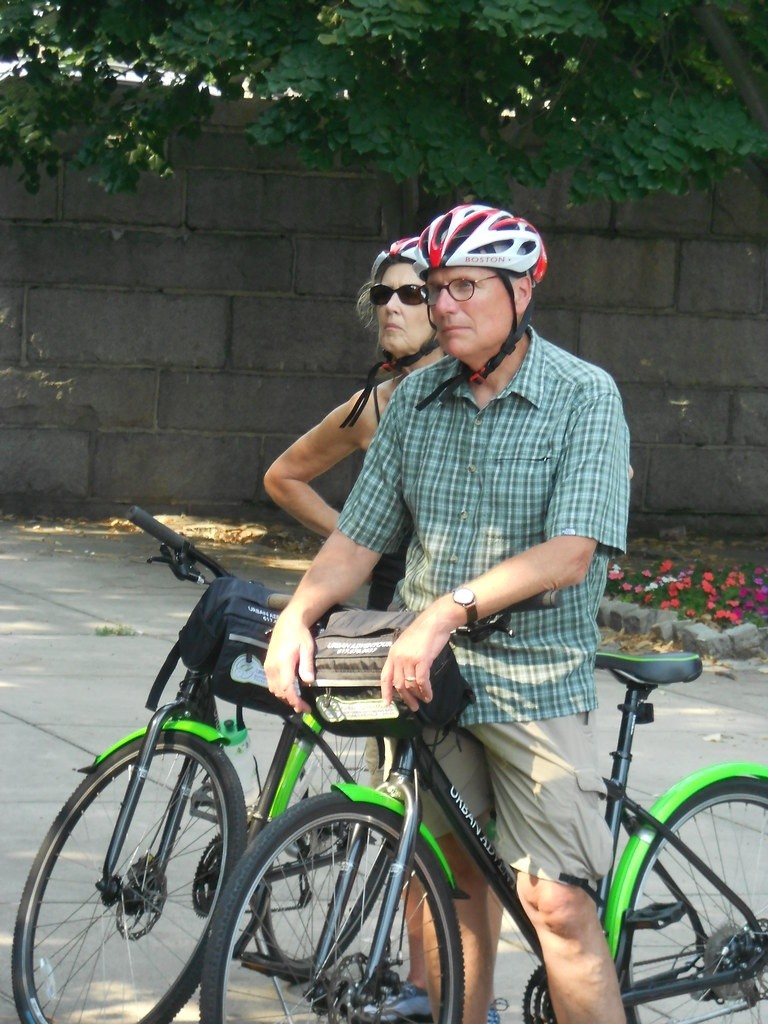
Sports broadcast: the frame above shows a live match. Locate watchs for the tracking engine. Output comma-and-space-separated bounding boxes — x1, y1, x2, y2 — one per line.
452, 586, 478, 627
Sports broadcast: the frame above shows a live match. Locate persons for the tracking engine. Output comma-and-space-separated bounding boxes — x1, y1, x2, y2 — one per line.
264, 237, 507, 1024
265, 205, 633, 1024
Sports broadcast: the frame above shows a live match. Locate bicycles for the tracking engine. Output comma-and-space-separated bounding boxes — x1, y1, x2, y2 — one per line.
197, 587, 768, 1024
9, 504, 394, 1024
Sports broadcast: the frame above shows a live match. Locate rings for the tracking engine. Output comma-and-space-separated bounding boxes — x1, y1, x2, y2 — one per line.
404, 677, 415, 681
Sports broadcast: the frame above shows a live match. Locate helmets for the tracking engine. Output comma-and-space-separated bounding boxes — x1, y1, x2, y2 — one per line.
370, 235, 420, 285
414, 203, 548, 288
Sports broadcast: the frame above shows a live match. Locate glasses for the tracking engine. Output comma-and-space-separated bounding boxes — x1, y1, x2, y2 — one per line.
368, 284, 430, 305
413, 275, 500, 306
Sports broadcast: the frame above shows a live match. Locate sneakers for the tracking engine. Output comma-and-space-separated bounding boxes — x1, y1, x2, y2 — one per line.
354, 980, 432, 1024
